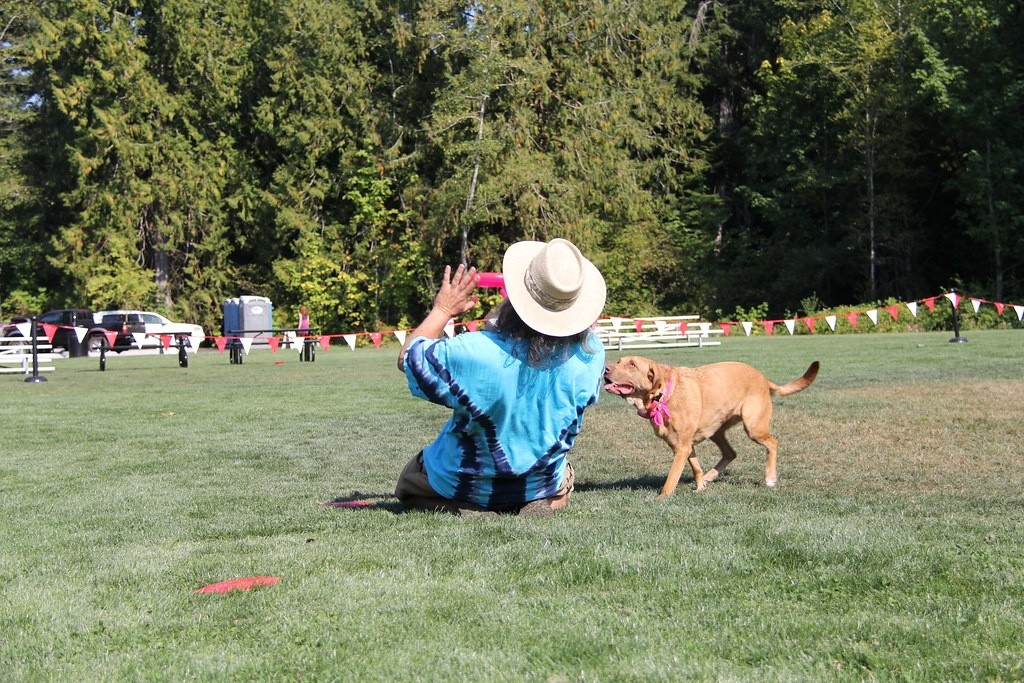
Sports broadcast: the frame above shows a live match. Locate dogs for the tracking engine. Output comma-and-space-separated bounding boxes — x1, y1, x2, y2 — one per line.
601, 355, 822, 498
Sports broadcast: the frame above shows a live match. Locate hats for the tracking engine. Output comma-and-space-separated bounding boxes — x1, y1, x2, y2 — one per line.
502, 238, 607, 337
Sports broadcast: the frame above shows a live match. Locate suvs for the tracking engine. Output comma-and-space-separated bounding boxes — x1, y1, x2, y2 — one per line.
93, 311, 206, 354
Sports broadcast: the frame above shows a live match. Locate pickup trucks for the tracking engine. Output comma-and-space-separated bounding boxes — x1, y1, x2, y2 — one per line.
2, 309, 134, 354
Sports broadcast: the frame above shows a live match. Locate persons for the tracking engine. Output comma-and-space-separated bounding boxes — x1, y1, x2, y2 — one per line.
396, 238, 607, 513
297, 306, 309, 337
